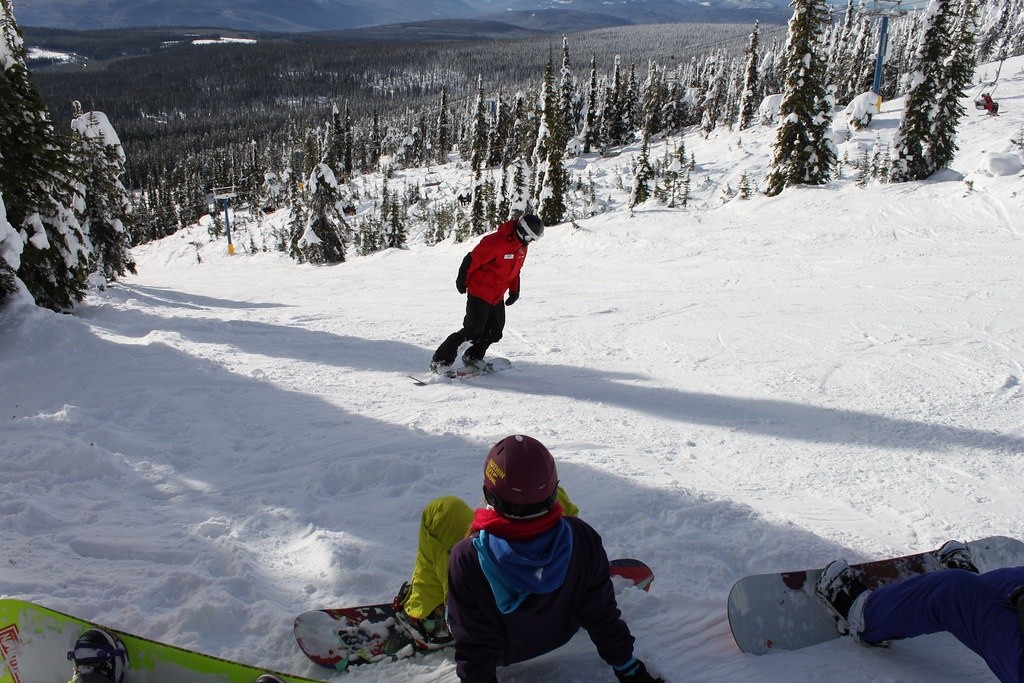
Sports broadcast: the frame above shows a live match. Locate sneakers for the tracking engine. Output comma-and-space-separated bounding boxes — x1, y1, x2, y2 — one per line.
73, 628, 123, 683
256, 674, 285, 683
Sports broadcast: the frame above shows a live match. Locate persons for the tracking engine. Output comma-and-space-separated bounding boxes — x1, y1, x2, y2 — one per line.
818, 539, 1023, 683
396, 433, 667, 683
429, 214, 545, 381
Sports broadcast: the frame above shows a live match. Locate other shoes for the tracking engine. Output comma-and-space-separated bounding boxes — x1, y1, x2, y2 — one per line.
819, 562, 891, 647
392, 581, 447, 641
462, 353, 493, 371
938, 539, 979, 574
430, 361, 456, 378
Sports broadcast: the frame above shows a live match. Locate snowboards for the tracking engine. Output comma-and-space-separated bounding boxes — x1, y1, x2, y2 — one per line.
0, 600, 328, 683
727, 535, 1024, 658
407, 356, 514, 385
292, 556, 656, 668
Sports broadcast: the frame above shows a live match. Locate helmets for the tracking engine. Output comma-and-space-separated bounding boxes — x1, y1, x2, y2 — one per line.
516, 215, 544, 245
484, 435, 559, 517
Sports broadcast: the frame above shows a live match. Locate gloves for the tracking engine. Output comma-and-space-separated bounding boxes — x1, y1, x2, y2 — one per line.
505, 292, 519, 306
613, 659, 664, 683
456, 275, 467, 294
